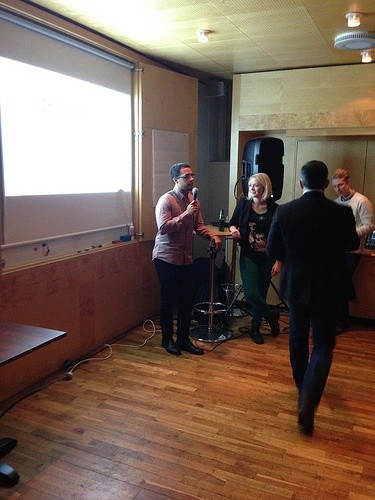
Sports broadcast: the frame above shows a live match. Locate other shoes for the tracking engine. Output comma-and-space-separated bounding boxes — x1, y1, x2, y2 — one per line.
269, 310, 280, 337
250, 329, 264, 344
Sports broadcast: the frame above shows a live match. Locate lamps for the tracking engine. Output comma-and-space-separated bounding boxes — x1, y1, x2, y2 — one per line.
197, 30, 212, 42
345, 13, 363, 27
333, 32, 375, 50
360, 51, 372, 63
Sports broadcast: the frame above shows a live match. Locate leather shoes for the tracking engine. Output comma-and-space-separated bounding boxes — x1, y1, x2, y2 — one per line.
162, 336, 181, 355
176, 337, 203, 355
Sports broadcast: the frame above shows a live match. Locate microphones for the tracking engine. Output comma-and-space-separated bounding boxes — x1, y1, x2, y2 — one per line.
192, 186, 199, 201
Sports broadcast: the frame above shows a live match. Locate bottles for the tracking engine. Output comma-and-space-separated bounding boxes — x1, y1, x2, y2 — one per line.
219, 209, 225, 232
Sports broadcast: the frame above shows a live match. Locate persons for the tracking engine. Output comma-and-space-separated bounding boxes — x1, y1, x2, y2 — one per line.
228, 173, 280, 345
265, 160, 360, 435
152, 162, 223, 355
332, 169, 375, 336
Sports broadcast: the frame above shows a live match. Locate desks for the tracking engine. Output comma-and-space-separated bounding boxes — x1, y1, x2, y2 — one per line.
191, 225, 232, 343
0, 320, 67, 486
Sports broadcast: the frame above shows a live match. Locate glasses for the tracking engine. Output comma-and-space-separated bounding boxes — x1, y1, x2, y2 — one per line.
178, 173, 196, 179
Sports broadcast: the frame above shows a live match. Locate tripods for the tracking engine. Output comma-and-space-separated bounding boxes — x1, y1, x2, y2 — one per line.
225, 274, 290, 320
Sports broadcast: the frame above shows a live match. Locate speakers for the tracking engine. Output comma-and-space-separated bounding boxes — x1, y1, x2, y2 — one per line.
242, 136, 285, 201
